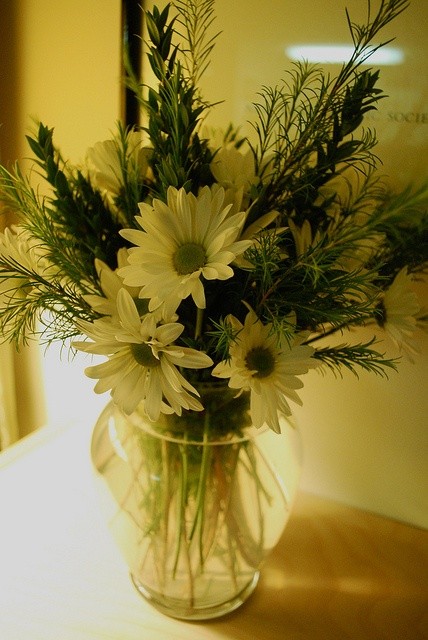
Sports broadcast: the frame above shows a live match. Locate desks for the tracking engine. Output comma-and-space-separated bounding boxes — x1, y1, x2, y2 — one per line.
0, 416, 426, 639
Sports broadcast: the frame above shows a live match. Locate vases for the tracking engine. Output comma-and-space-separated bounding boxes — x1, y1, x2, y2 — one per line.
91, 385, 299, 625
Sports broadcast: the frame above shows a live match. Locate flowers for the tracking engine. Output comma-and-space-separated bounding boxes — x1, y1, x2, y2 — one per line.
0, 0, 428, 609
